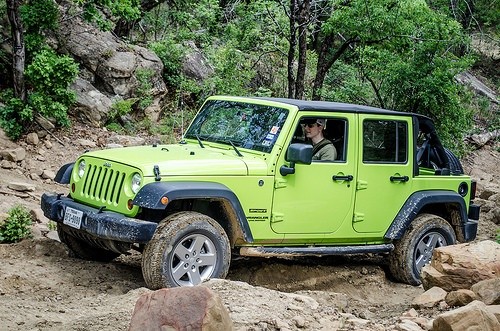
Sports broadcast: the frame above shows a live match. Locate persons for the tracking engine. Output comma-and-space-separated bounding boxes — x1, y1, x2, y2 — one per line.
297, 116, 339, 161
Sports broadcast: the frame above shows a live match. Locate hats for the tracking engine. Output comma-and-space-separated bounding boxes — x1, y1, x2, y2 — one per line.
300, 118, 325, 126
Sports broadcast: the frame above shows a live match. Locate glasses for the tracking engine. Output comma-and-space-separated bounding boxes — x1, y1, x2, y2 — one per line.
304, 124, 317, 128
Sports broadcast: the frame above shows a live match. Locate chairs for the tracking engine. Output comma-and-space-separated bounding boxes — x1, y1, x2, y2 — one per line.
325, 119, 344, 160
385, 127, 405, 160
294, 126, 304, 143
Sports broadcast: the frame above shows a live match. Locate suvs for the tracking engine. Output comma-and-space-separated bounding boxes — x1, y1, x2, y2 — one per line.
40, 94, 482, 292
